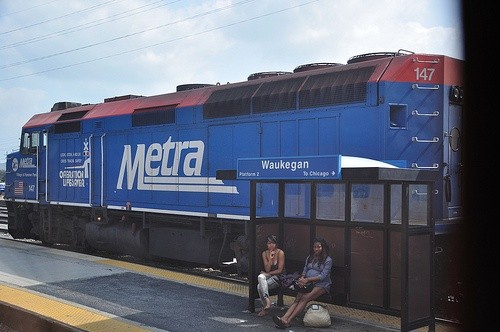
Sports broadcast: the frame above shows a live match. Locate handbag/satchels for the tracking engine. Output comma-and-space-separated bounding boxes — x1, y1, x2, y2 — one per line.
303, 304, 331, 327
294, 281, 313, 292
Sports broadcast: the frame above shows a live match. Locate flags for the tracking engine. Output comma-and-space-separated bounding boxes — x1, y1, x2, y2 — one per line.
13, 180, 23, 196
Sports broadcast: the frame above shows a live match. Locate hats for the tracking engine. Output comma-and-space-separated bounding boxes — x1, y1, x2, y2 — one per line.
267, 234, 276, 242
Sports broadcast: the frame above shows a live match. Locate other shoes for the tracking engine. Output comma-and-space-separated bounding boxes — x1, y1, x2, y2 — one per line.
256, 310, 268, 317
264, 301, 271, 311
272, 315, 291, 329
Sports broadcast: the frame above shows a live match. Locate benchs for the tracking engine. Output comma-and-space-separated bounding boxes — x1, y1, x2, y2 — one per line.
268, 260, 350, 303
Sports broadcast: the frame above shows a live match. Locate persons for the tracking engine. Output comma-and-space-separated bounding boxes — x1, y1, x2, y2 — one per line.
273, 239, 332, 328
257, 234, 288, 317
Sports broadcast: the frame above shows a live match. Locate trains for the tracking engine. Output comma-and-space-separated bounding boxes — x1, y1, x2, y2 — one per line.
2, 48, 465, 277
